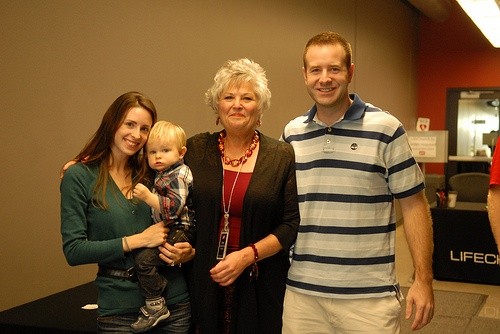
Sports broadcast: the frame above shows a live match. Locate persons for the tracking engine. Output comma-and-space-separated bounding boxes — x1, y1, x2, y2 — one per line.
278, 32, 435, 334
60, 91, 197, 334
61, 58, 300, 334
128, 121, 198, 332
486, 134, 499, 251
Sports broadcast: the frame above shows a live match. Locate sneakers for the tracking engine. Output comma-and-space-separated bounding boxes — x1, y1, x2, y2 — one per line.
129, 302, 170, 332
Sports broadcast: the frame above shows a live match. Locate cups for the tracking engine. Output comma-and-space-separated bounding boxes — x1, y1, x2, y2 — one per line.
447, 191, 458, 208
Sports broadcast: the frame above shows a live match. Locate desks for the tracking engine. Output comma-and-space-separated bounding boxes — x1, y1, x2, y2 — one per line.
429, 199, 500, 286
0, 278, 99, 334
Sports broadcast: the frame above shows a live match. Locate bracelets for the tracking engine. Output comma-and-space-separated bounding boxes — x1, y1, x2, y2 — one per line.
249, 243, 259, 263
124, 236, 132, 252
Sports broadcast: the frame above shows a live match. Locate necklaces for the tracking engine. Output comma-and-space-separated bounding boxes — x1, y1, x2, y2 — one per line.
217, 130, 260, 167
214, 140, 254, 259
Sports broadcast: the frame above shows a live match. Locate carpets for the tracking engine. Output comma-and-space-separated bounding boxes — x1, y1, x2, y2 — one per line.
399, 285, 500, 334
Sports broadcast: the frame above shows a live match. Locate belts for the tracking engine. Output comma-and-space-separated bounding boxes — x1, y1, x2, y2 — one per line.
97, 266, 139, 279
